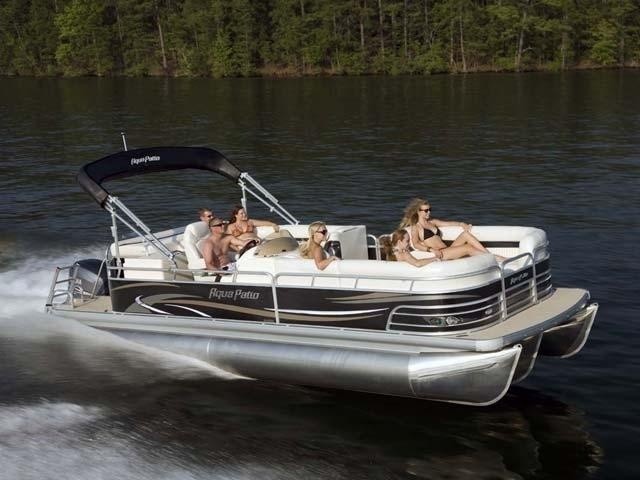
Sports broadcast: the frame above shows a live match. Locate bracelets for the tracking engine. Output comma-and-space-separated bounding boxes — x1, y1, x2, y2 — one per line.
427, 247, 432, 252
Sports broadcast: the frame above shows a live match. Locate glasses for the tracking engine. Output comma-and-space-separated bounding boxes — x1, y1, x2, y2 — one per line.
211, 223, 225, 226
202, 213, 214, 219
317, 230, 327, 236
419, 207, 431, 214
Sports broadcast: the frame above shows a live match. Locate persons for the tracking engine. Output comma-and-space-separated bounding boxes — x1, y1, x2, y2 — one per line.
299, 221, 340, 270
202, 217, 252, 276
382, 229, 441, 266
199, 207, 214, 222
399, 197, 506, 262
225, 206, 279, 253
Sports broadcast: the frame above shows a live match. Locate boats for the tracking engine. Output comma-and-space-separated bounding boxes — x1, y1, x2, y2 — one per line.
46, 130, 599, 408
